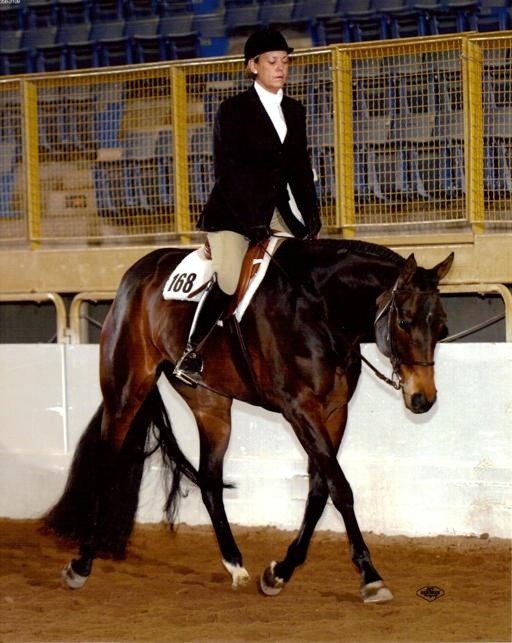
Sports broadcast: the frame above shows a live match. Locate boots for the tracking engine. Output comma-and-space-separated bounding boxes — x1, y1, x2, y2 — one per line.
175, 272, 237, 388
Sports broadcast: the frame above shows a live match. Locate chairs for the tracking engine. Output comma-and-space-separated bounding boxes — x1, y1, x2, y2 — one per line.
0, 0, 512, 219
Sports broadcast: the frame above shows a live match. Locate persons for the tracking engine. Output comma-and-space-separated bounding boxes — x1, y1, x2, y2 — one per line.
173, 27, 323, 385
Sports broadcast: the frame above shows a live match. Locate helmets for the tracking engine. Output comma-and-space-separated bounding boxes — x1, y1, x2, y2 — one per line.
244, 29, 293, 63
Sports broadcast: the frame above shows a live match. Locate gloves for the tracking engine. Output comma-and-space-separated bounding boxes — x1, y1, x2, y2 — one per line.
247, 225, 270, 241
307, 212, 322, 238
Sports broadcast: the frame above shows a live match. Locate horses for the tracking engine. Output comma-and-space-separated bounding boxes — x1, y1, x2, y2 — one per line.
35, 238, 455, 605
